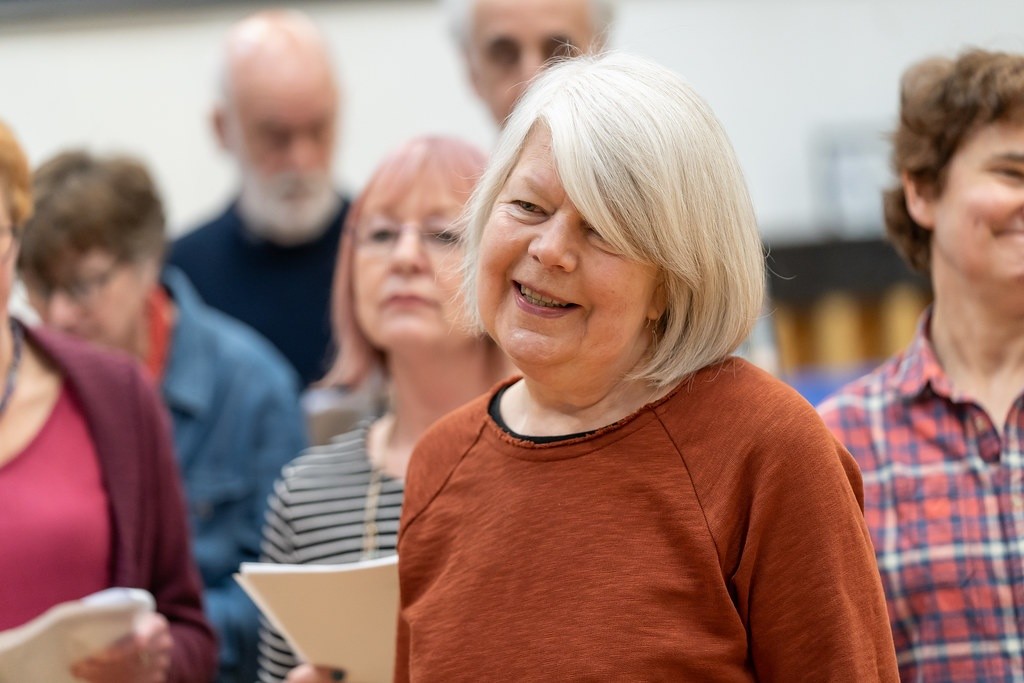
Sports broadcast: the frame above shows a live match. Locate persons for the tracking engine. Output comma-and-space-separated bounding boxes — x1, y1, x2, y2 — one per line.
12, 151, 312, 682
165, 8, 357, 403
2, 121, 219, 682
813, 45, 1024, 682
444, 1, 620, 133
230, 136, 517, 680
393, 54, 901, 683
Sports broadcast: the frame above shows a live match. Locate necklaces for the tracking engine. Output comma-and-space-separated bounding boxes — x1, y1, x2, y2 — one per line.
362, 410, 395, 561
0, 314, 25, 410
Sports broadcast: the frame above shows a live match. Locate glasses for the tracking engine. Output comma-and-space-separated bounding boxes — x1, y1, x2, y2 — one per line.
361, 227, 460, 249
17, 250, 141, 301
